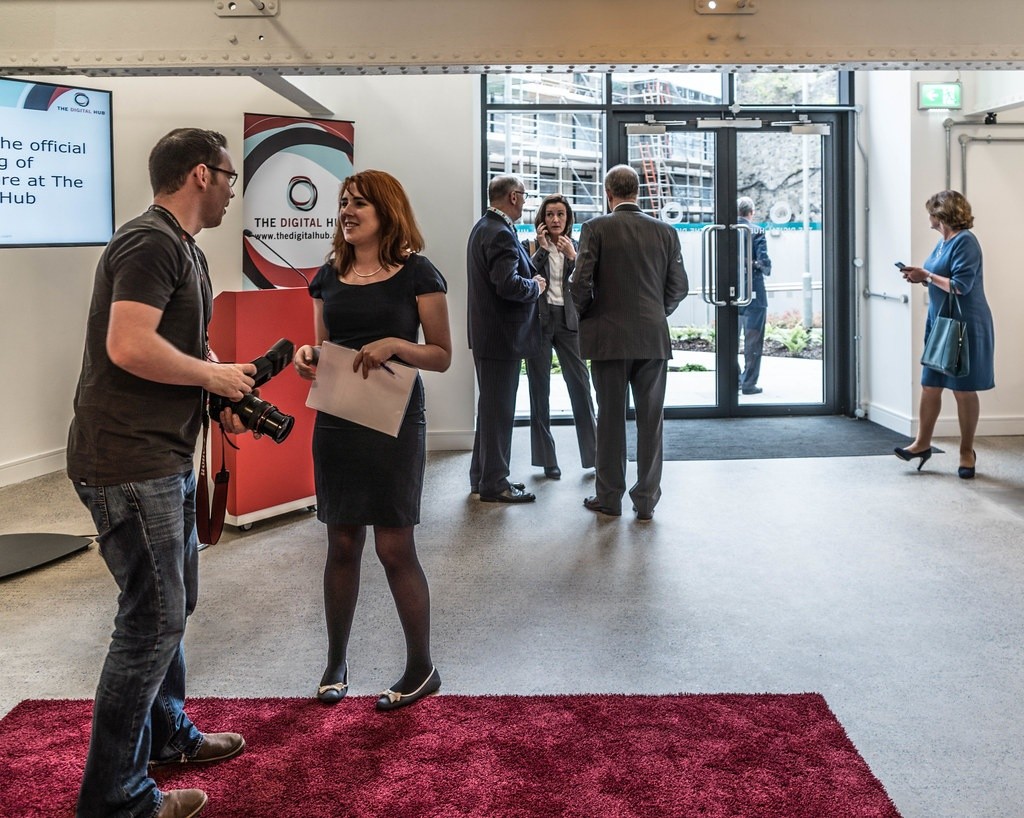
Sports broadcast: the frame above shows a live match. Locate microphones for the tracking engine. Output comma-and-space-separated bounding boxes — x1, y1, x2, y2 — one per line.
243, 228, 309, 286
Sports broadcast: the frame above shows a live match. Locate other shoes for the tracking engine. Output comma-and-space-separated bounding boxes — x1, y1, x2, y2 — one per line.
583, 496, 621, 516
637, 509, 655, 522
742, 385, 762, 394
162, 732, 246, 763
317, 659, 349, 703
156, 788, 206, 818
376, 665, 441, 710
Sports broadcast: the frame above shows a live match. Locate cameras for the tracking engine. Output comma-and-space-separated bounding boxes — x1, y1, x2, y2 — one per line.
212, 337, 296, 444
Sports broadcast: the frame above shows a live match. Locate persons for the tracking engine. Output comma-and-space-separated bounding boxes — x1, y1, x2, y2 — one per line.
521, 194, 597, 478
569, 164, 689, 519
894, 190, 996, 479
292, 170, 452, 710
66, 128, 260, 818
737, 197, 772, 395
467, 176, 546, 503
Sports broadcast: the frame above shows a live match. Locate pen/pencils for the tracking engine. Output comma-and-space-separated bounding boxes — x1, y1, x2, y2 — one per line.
381, 363, 395, 375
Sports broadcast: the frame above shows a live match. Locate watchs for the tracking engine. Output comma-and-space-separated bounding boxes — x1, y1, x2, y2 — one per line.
926, 273, 933, 283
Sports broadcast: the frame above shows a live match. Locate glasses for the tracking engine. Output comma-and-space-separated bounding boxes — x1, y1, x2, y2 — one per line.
194, 165, 238, 187
509, 191, 528, 199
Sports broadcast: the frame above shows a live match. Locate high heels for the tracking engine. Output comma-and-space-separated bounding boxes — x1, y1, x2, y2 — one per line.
958, 450, 976, 479
894, 447, 932, 471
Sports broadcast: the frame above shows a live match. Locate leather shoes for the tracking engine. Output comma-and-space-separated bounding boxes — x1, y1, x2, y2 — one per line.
544, 465, 561, 479
471, 482, 525, 494
480, 485, 536, 502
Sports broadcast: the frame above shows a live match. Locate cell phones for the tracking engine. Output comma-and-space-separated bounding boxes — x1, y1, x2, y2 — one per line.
895, 262, 905, 269
542, 220, 549, 235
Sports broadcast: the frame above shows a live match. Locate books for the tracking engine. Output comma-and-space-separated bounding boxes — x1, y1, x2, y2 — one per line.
305, 341, 418, 438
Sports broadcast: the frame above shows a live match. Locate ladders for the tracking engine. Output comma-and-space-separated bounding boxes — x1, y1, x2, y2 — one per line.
642, 81, 672, 207
640, 142, 661, 219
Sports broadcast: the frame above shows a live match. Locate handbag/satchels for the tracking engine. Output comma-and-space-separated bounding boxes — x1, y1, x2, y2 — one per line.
920, 279, 970, 379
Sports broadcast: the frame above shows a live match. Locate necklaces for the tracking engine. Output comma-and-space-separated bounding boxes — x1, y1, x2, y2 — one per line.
352, 260, 386, 277
937, 232, 954, 257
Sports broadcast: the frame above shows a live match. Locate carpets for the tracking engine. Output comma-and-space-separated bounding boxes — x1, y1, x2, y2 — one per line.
597, 414, 946, 461
0, 692, 903, 818
